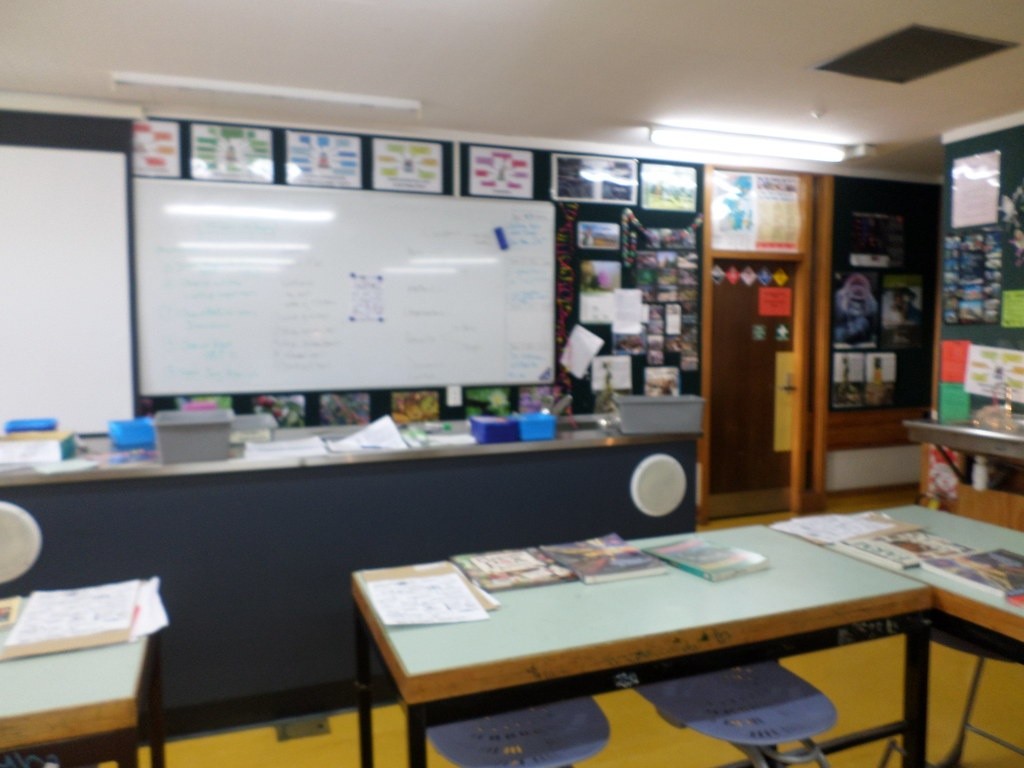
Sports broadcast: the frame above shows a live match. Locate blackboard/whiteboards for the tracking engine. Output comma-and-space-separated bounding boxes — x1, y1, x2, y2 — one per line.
127, 174, 563, 401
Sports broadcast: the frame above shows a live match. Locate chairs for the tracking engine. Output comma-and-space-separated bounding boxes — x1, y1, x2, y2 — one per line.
932, 485, 1024, 665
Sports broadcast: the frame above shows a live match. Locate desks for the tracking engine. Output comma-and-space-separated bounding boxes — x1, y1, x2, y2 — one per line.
351, 524, 935, 768
905, 416, 1024, 486
0, 412, 703, 741
1, 578, 165, 768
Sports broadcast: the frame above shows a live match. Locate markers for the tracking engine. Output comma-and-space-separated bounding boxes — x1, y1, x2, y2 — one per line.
539, 393, 574, 417
398, 421, 451, 449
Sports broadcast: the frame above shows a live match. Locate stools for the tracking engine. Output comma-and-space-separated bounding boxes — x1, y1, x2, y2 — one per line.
632, 661, 839, 768
771, 504, 1024, 768
425, 696, 612, 768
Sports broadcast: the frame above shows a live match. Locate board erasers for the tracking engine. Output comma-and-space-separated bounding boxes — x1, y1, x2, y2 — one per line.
3, 416, 55, 432
494, 226, 509, 250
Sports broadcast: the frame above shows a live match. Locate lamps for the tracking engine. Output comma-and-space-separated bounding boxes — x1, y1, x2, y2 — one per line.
640, 120, 847, 168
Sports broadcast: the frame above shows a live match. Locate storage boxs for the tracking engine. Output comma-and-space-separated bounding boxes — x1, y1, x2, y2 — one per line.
470, 416, 519, 444
511, 412, 557, 440
612, 394, 706, 433
154, 410, 232, 464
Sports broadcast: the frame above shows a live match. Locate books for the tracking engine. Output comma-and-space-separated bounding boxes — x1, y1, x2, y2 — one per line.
641, 536, 767, 581
922, 547, 1024, 596
542, 531, 667, 583
450, 545, 581, 592
0, 577, 145, 663
837, 528, 977, 569
